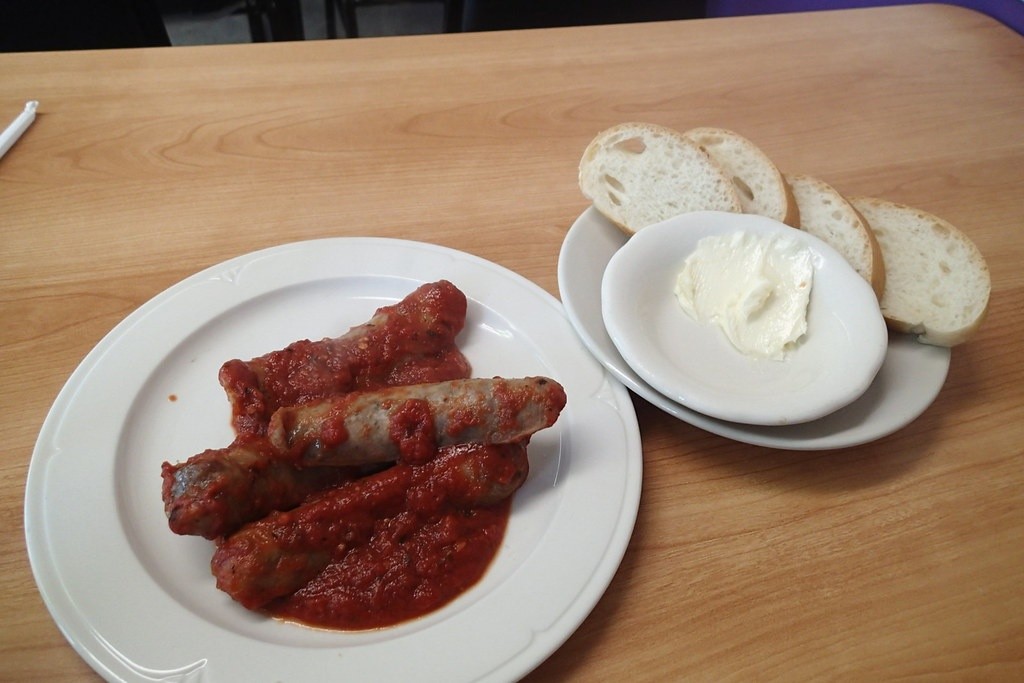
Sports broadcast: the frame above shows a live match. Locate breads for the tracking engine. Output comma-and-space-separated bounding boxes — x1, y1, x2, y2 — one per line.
576, 121, 994, 351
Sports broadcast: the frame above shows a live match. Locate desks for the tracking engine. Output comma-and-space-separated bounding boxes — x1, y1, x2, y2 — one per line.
0, 2, 1024, 683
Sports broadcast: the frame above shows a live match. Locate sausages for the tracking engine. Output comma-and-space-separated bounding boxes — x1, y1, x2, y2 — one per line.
160, 277, 567, 612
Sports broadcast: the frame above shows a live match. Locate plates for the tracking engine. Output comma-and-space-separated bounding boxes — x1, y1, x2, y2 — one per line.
22, 238, 642, 683
556, 197, 953, 450
598, 210, 887, 424
